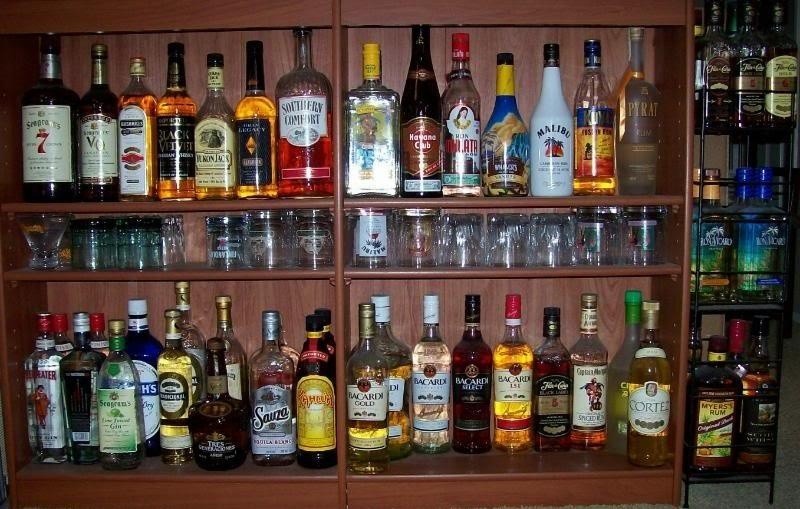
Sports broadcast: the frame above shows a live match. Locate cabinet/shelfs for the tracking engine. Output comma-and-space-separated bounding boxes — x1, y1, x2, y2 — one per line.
249, 313, 299, 374
193, 53, 237, 202
492, 294, 534, 453
692, 168, 702, 208
572, 207, 623, 267
343, 208, 397, 268
452, 295, 495, 454
293, 209, 337, 271
58, 311, 107, 466
189, 337, 251, 471
567, 293, 608, 453
73, 43, 120, 202
574, 39, 618, 195
726, 166, 754, 210
51, 312, 74, 359
124, 298, 164, 459
347, 303, 390, 475
90, 313, 116, 359
398, 30, 441, 198
202, 215, 247, 271
728, 318, 751, 369
690, 169, 739, 304
482, 54, 530, 197
246, 210, 298, 270
237, 40, 278, 200
369, 292, 414, 462
532, 305, 572, 454
299, 313, 338, 470
617, 27, 664, 196
111, 57, 159, 202
696, 1, 734, 134
18, 33, 79, 204
627, 300, 671, 468
413, 295, 453, 454
767, 3, 800, 133
156, 309, 192, 466
275, 29, 338, 198
98, 318, 143, 471
116, 216, 166, 271
735, 2, 768, 134
174, 280, 205, 406
686, 333, 743, 479
213, 295, 250, 403
606, 290, 645, 458
342, 43, 401, 198
23, 313, 70, 464
735, 314, 780, 476
622, 206, 670, 265
155, 41, 197, 202
71, 218, 116, 271
530, 42, 575, 197
312, 307, 338, 373
250, 310, 298, 468
393, 209, 441, 269
441, 33, 485, 198
734, 167, 791, 307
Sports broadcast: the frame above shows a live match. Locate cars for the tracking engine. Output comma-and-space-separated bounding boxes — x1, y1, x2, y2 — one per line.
530, 213, 582, 268
72, 219, 118, 270
16, 215, 70, 270
486, 213, 529, 271
176, 213, 187, 268
292, 208, 334, 271
242, 208, 294, 269
127, 215, 164, 269
204, 215, 242, 270
345, 206, 396, 269
99, 214, 129, 269
161, 216, 176, 268
437, 213, 486, 270
391, 208, 437, 269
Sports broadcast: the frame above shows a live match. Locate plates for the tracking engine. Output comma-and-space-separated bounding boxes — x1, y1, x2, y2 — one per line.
682, 3, 794, 506
0, 0, 695, 506
